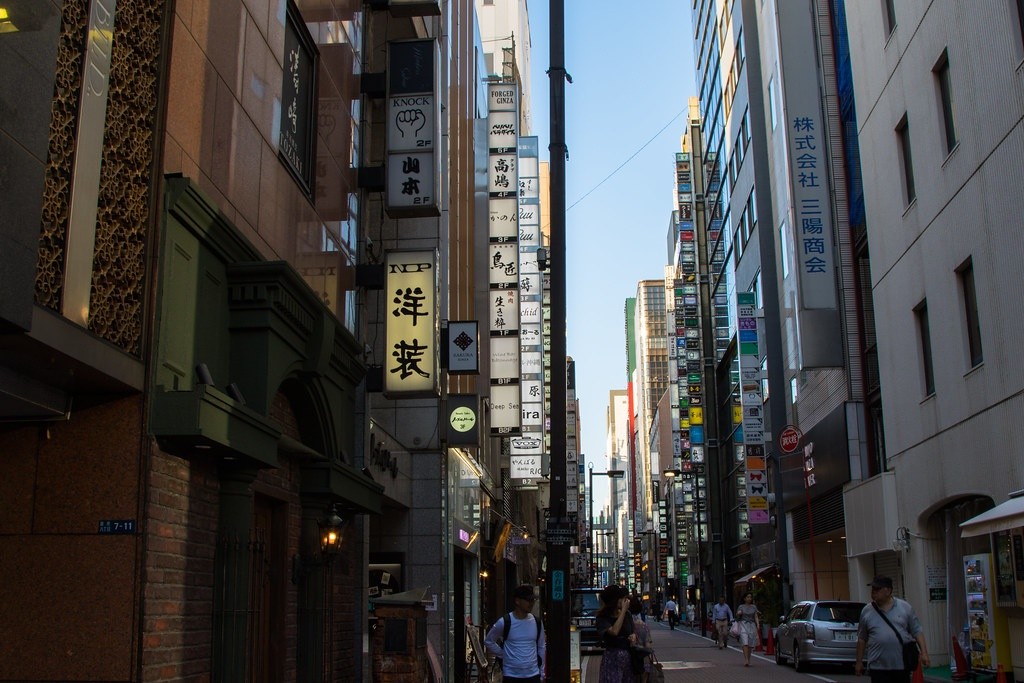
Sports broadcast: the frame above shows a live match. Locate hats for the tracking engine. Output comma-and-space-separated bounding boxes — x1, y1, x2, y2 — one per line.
601, 585, 628, 603
867, 575, 893, 588
515, 585, 541, 598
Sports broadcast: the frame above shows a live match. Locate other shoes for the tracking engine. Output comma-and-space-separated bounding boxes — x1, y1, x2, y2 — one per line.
724, 640, 728, 648
720, 646, 723, 649
744, 662, 752, 666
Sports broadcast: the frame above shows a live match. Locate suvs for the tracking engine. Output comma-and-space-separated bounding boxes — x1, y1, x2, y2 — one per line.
568, 586, 610, 646
774, 600, 869, 673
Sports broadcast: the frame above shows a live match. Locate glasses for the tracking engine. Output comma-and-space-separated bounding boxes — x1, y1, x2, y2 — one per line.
520, 597, 537, 602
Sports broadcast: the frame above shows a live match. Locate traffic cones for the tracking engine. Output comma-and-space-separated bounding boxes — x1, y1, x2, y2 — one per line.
752, 624, 765, 652
911, 654, 924, 683
950, 636, 972, 679
763, 628, 776, 655
996, 663, 1008, 683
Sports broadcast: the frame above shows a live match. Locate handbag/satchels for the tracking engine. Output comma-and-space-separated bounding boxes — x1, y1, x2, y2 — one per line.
902, 640, 919, 672
647, 651, 665, 683
630, 645, 652, 673
711, 623, 718, 640
729, 613, 741, 638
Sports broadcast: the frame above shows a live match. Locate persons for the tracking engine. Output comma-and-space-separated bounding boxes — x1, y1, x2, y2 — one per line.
653, 601, 661, 622
640, 598, 645, 622
595, 585, 638, 683
482, 585, 546, 683
627, 595, 655, 683
712, 595, 733, 650
736, 591, 759, 666
686, 601, 696, 631
855, 574, 931, 683
661, 596, 676, 632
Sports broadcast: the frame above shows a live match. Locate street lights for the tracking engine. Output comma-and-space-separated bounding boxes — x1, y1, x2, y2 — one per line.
594, 530, 615, 589
663, 466, 709, 638
588, 467, 625, 589
637, 531, 661, 622
